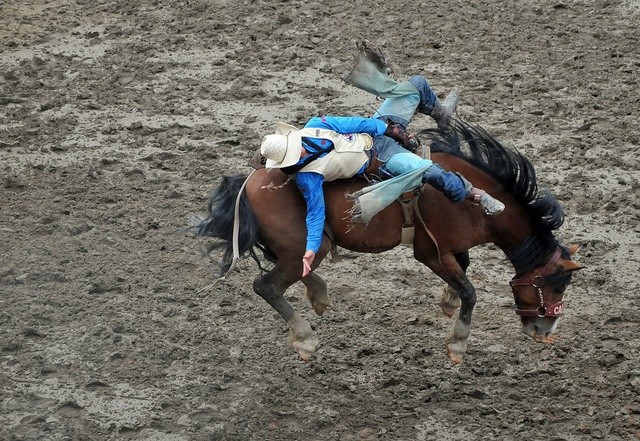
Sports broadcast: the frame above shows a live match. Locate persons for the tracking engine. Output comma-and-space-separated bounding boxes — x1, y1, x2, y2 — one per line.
260, 75, 506, 278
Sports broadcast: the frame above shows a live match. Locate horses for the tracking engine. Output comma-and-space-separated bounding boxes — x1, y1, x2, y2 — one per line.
195, 112, 585, 366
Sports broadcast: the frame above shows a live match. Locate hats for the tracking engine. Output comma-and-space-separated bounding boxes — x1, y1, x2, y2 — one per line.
260, 122, 302, 169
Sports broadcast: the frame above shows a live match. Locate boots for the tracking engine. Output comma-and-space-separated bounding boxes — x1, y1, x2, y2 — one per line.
455, 172, 506, 216
430, 88, 459, 130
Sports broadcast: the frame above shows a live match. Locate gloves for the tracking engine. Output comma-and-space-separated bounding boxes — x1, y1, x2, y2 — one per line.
384, 124, 421, 149
